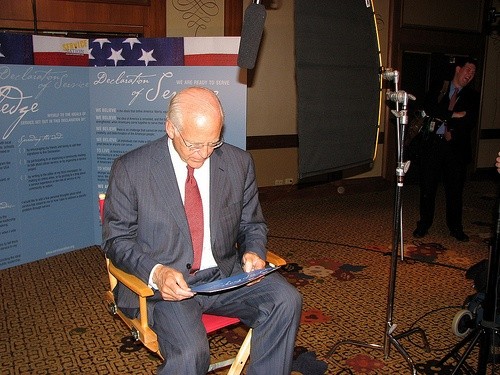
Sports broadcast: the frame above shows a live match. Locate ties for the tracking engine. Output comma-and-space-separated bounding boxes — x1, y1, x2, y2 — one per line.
444, 87, 459, 141
183, 164, 204, 278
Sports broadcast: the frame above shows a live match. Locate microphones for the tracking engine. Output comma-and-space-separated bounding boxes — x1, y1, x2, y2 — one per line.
236, 0, 266, 69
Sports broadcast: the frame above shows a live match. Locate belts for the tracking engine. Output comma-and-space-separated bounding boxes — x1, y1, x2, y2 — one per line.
436, 134, 446, 140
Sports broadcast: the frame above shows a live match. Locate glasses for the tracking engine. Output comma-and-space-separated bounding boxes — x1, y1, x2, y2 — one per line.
167, 119, 225, 149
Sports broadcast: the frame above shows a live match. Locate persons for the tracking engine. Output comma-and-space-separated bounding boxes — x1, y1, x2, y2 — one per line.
495, 152, 500, 174
413, 59, 478, 242
102, 87, 303, 375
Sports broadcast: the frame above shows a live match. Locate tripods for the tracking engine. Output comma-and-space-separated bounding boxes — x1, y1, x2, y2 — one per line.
325, 65, 433, 375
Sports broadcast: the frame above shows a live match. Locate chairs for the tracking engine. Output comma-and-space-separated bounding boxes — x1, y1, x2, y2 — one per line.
99, 194, 287, 375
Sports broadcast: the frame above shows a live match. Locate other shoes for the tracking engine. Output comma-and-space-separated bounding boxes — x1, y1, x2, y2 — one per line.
449, 230, 469, 242
413, 224, 430, 237
